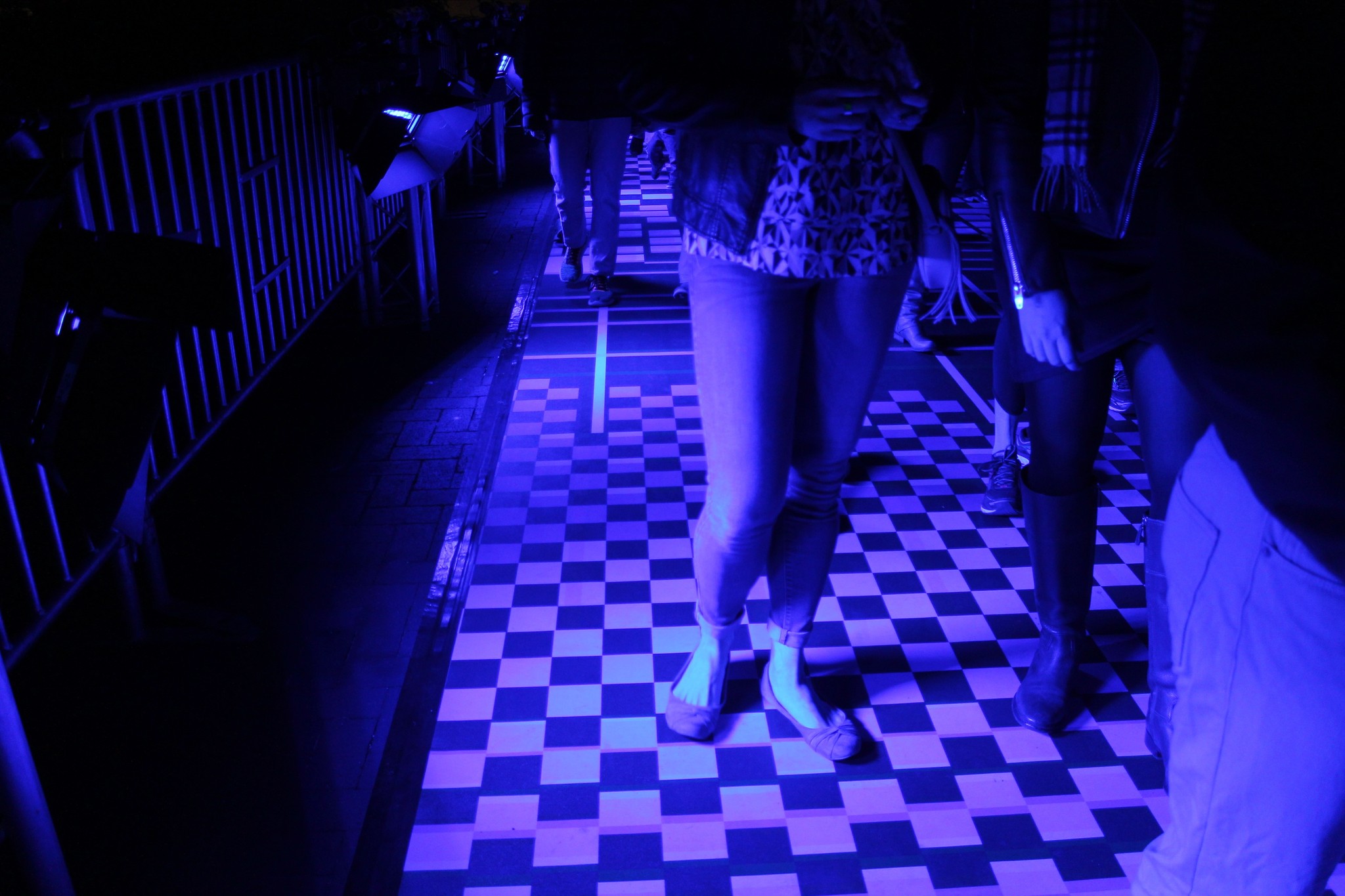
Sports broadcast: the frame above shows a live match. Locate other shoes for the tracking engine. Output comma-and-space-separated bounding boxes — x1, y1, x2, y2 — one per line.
761, 661, 863, 761
665, 642, 730, 742
674, 284, 689, 300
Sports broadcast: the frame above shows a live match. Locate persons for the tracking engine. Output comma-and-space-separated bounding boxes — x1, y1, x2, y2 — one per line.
629, 132, 645, 155
673, 243, 689, 299
892, 192, 965, 353
1109, 353, 1137, 413
507, 0, 642, 306
633, 0, 973, 762
964, 159, 990, 206
1129, 0, 1345, 896
979, 225, 1031, 518
972, 1, 1239, 793
644, 128, 678, 189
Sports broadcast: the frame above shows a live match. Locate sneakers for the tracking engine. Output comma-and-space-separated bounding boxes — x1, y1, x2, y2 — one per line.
559, 235, 588, 284
587, 273, 616, 306
1015, 426, 1031, 465
1108, 370, 1136, 415
980, 450, 1024, 516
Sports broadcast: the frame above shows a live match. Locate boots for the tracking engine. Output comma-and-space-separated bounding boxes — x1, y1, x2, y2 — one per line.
1134, 515, 1178, 800
1010, 464, 1102, 733
893, 289, 936, 352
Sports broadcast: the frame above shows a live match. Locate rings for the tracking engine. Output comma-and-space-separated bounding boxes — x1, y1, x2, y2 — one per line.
843, 101, 852, 115
901, 108, 915, 121
